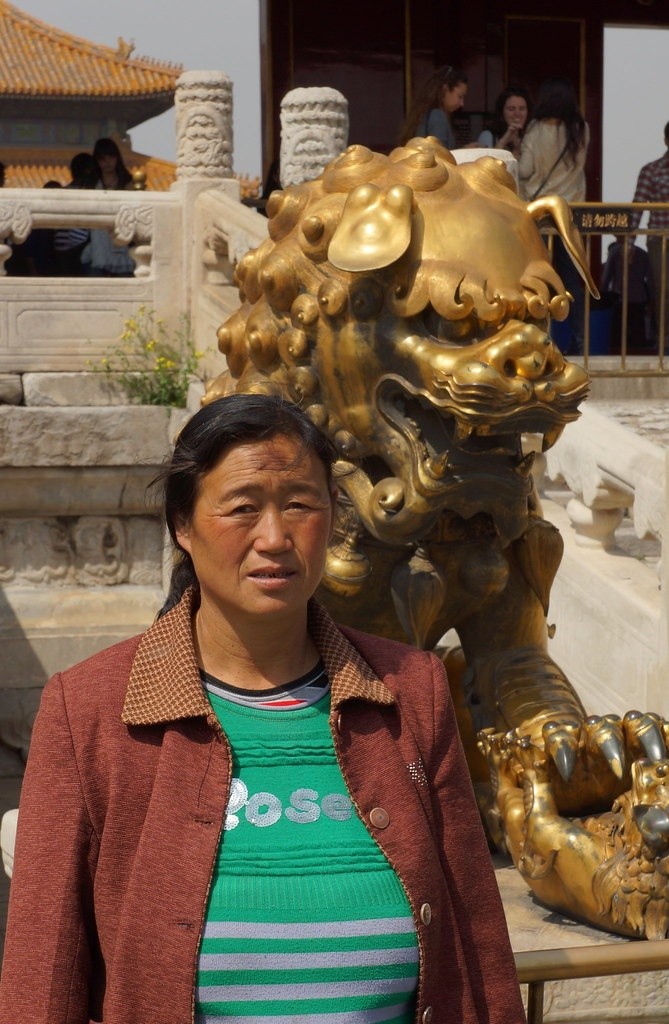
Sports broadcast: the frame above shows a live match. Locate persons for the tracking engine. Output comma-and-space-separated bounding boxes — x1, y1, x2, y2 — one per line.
3, 138, 137, 277
598, 233, 649, 356
397, 65, 590, 355
622, 122, 669, 356
0, 393, 528, 1024
